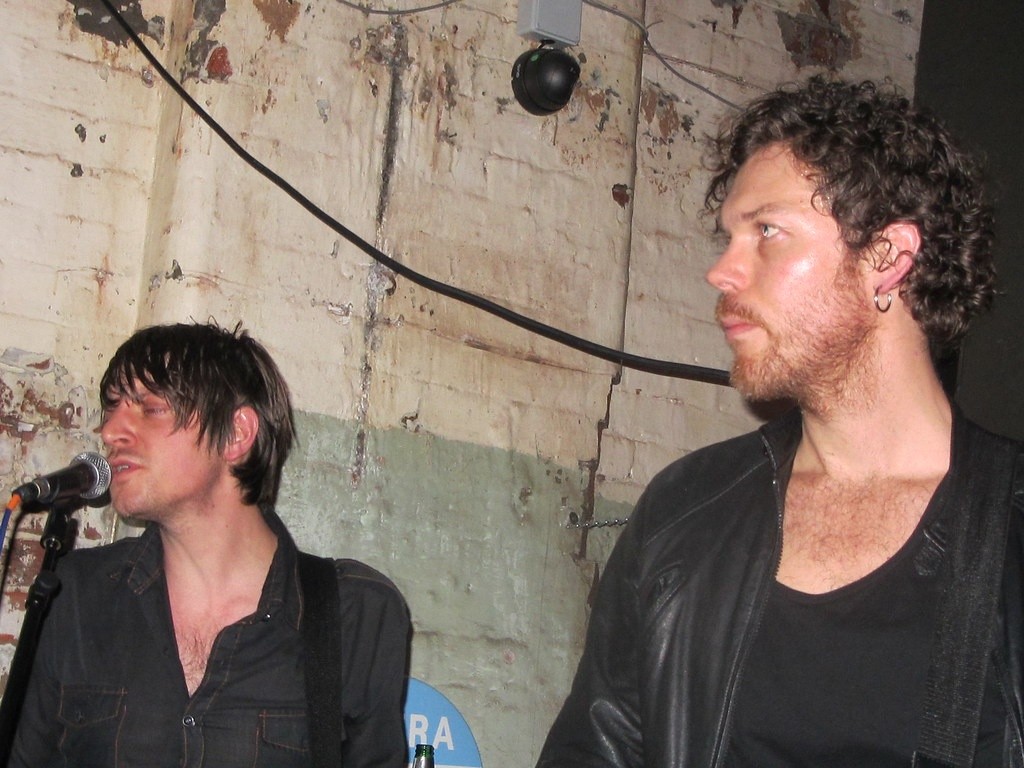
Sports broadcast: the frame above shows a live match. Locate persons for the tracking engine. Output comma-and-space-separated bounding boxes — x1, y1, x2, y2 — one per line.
0, 323, 415, 768
535, 74, 1024, 768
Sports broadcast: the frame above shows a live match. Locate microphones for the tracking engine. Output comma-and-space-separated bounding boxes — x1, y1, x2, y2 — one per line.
11, 451, 112, 505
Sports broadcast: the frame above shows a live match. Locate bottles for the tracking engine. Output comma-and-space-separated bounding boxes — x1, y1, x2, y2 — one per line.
412, 744, 435, 768
